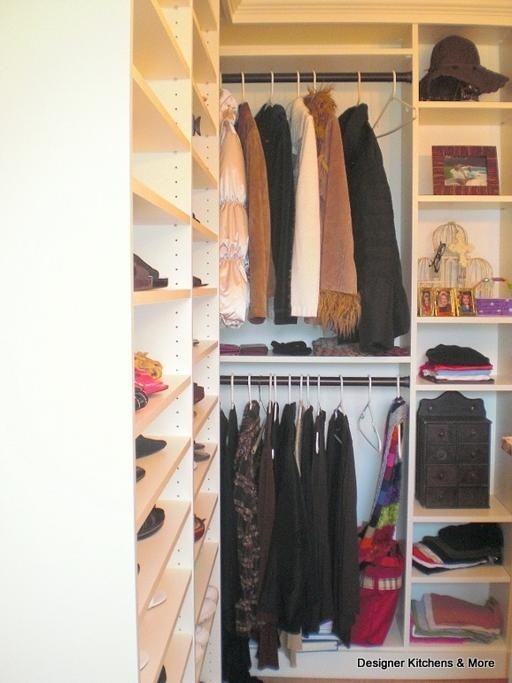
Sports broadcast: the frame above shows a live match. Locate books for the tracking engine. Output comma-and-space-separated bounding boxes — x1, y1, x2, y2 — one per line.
298, 618, 341, 652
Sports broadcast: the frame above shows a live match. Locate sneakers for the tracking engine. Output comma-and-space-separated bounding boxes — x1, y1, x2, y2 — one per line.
193, 586, 217, 662
137, 508, 164, 539
136, 435, 167, 458
135, 351, 168, 410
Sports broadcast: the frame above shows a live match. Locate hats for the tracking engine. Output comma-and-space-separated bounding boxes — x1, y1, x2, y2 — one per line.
419, 36, 508, 101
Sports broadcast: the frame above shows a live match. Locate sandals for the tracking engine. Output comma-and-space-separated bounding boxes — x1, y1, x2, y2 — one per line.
193, 277, 208, 286
134, 254, 167, 291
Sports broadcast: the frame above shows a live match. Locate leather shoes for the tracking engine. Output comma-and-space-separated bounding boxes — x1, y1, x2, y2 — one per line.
194, 441, 210, 462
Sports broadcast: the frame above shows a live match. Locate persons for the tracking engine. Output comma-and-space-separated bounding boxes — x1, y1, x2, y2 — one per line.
458, 294, 473, 312
421, 290, 432, 316
437, 291, 450, 313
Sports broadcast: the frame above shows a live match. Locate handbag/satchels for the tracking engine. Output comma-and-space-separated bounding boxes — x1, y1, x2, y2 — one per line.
351, 527, 404, 647
